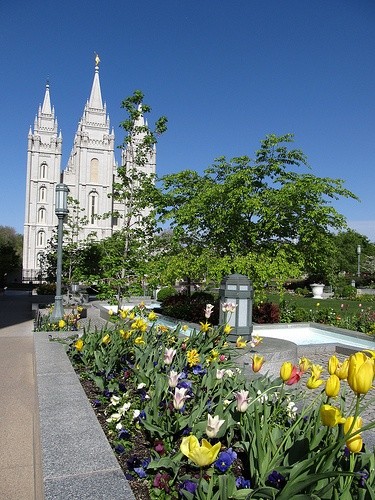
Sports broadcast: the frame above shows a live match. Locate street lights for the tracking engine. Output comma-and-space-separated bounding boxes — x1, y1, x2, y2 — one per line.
217, 274, 255, 344
50, 183, 70, 325
356, 244, 363, 278
